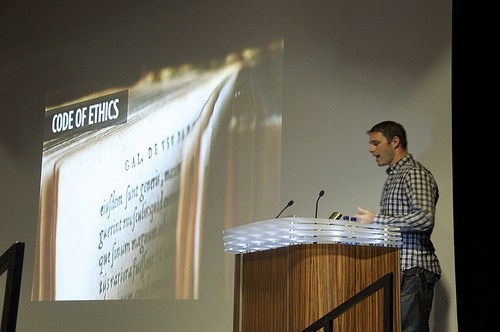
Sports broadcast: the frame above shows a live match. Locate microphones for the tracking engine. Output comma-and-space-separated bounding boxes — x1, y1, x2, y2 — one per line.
276, 200, 294, 218
313, 190, 325, 244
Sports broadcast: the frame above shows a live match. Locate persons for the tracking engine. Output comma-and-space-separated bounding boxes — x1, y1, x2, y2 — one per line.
351, 121, 442, 332
